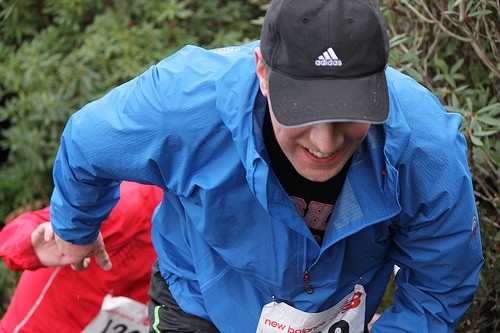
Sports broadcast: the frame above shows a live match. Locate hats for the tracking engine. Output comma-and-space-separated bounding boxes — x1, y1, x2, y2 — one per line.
261, 0, 390, 127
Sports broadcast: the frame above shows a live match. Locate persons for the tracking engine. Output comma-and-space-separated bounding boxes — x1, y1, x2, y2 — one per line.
50, 0, 486, 333
0, 181, 163, 333
